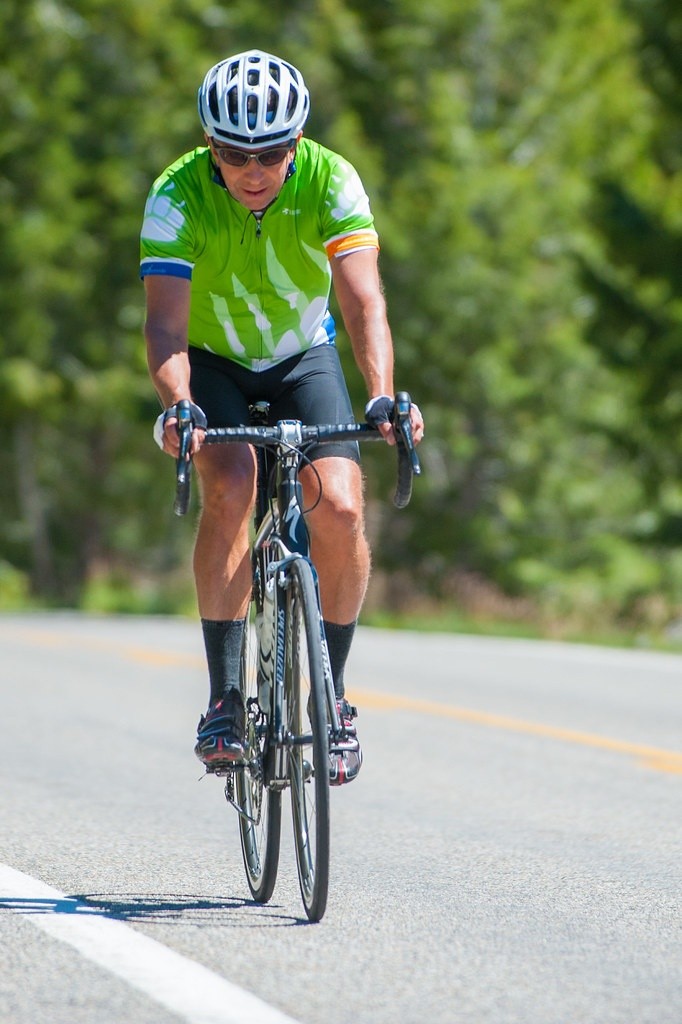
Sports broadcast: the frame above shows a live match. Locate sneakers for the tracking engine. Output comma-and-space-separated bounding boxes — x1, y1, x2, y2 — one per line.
306, 689, 362, 786
198, 699, 245, 766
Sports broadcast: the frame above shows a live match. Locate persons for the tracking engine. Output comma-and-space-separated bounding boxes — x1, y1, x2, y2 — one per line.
140, 50, 424, 786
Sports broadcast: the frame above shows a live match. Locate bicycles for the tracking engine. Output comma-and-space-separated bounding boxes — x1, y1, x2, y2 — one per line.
173, 385, 426, 922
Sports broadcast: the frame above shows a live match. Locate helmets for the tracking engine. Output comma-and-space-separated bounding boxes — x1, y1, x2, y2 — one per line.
197, 48, 310, 150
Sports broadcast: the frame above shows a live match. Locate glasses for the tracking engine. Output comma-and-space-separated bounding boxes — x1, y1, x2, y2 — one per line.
204, 134, 297, 166
